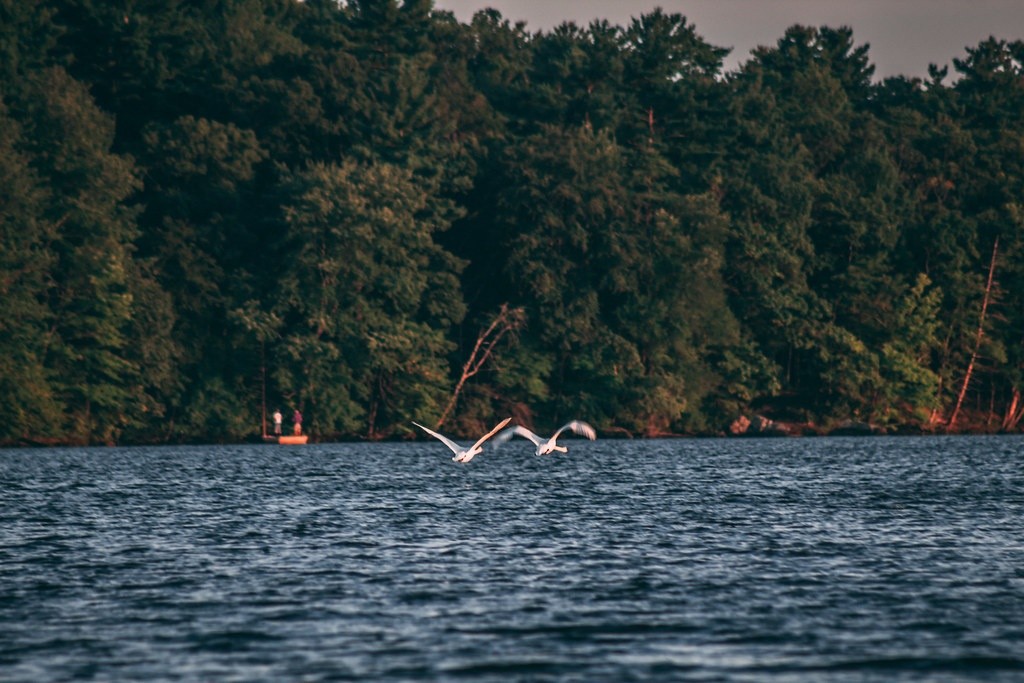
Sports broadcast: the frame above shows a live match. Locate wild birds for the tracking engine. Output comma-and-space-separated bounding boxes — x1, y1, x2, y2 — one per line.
410, 417, 597, 464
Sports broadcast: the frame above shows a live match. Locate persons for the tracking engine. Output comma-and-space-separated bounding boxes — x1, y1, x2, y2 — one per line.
271, 408, 283, 437
291, 410, 303, 435
